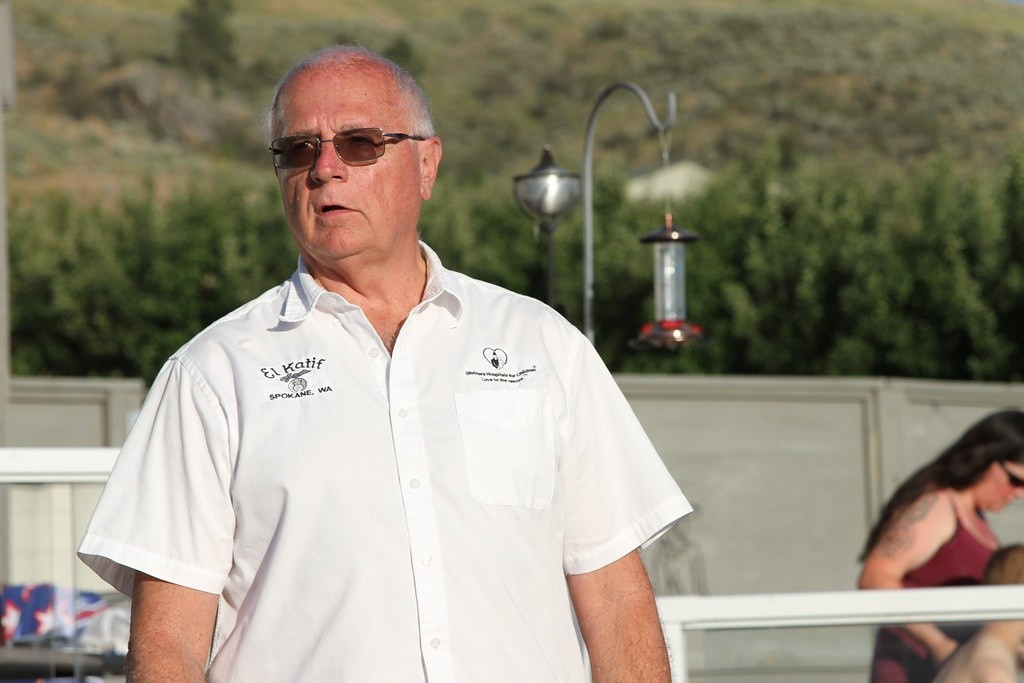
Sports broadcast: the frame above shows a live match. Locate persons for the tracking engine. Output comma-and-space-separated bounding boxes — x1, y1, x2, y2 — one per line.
76, 46, 694, 683
856, 409, 1024, 683
932, 544, 1024, 683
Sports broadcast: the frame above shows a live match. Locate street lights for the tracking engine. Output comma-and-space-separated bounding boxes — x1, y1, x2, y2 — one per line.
511, 144, 582, 300
583, 82, 706, 357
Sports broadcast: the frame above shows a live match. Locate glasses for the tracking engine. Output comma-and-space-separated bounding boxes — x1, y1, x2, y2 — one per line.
269, 128, 426, 169
999, 462, 1024, 487
